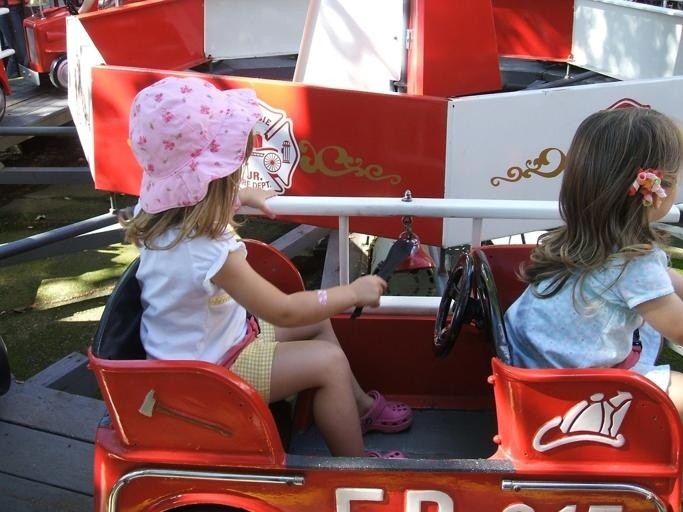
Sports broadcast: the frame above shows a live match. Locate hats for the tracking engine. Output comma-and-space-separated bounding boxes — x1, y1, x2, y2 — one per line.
127, 77, 261, 214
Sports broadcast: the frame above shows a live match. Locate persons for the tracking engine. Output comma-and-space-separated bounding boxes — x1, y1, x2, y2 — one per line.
0, 0, 47, 77
77, 0, 122, 15
500, 106, 683, 422
117, 76, 414, 457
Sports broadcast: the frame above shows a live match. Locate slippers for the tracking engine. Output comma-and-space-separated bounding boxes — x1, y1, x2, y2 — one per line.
359, 390, 414, 433
365, 449, 404, 459
